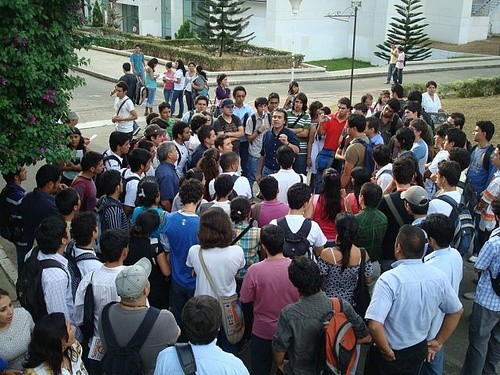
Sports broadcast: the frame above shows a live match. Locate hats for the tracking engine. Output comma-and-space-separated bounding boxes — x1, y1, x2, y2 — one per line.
115, 258, 150, 298
221, 98, 234, 108
143, 124, 167, 137
400, 186, 429, 206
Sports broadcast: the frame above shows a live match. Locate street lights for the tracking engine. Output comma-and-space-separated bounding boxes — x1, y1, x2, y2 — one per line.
289, 0, 302, 82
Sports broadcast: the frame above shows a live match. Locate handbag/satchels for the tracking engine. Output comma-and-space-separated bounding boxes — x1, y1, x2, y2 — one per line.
209, 105, 221, 118
78, 270, 94, 335
351, 246, 371, 328
218, 294, 245, 343
194, 77, 209, 96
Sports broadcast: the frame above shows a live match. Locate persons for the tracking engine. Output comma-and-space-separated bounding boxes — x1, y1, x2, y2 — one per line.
60, 111, 91, 187
110, 62, 141, 136
144, 58, 160, 116
384, 42, 405, 86
307, 80, 500, 195
162, 59, 231, 118
0, 158, 251, 375
71, 82, 311, 216
130, 45, 146, 84
241, 175, 500, 375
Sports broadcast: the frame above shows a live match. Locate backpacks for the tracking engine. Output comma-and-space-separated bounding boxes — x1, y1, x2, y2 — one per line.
15, 246, 69, 324
63, 240, 100, 303
321, 296, 360, 375
92, 151, 142, 253
97, 301, 159, 375
0, 189, 21, 243
135, 76, 147, 105
212, 175, 241, 201
277, 216, 312, 259
352, 140, 376, 176
438, 193, 474, 257
488, 232, 500, 296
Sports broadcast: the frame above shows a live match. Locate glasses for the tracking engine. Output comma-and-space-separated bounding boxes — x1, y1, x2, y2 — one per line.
447, 120, 456, 126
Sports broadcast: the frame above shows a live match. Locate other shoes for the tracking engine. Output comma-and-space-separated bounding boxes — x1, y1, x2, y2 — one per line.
464, 292, 477, 299
142, 111, 183, 119
469, 255, 479, 263
133, 126, 141, 136
